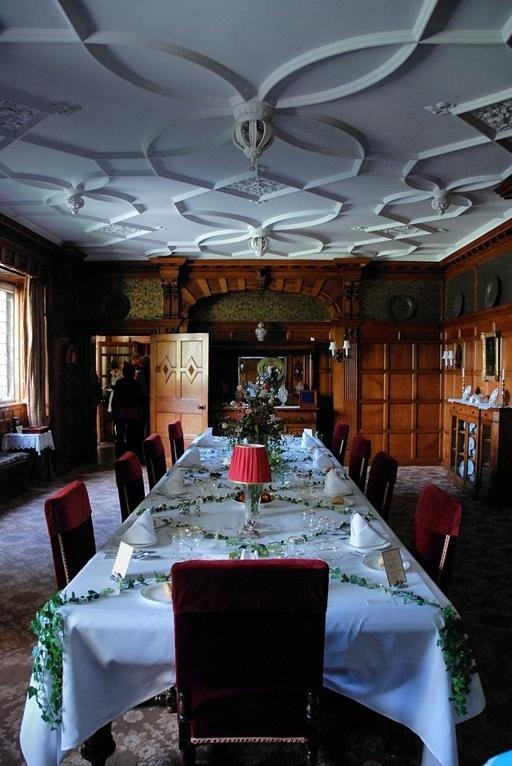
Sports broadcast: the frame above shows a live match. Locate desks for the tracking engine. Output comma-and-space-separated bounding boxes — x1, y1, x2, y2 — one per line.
1, 429, 55, 485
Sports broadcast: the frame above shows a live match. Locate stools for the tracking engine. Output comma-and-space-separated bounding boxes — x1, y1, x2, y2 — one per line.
0, 452, 32, 504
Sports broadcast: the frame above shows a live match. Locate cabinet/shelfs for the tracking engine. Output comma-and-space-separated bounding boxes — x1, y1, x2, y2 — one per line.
444, 399, 511, 503
213, 340, 319, 437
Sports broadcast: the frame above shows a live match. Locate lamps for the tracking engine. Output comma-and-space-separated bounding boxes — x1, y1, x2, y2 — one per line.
441, 350, 455, 374
329, 340, 352, 361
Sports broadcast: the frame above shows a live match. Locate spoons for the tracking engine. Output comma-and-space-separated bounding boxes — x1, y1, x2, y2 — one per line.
103, 550, 158, 561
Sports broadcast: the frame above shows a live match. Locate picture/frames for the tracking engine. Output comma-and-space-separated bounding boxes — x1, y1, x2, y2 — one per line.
298, 388, 317, 408
481, 331, 500, 382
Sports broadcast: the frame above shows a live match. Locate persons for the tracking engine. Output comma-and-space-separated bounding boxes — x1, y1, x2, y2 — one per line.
95, 351, 151, 465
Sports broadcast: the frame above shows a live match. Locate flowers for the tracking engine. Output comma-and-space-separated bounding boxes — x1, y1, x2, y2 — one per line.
219, 379, 285, 448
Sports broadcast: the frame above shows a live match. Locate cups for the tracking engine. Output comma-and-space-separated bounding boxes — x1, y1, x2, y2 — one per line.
178, 436, 352, 569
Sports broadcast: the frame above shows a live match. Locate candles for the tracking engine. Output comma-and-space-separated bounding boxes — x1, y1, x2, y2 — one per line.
502, 368, 504, 380
461, 367, 465, 377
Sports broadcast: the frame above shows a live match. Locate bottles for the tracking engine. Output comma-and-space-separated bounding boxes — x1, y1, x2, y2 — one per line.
255, 322, 267, 342
13, 417, 21, 433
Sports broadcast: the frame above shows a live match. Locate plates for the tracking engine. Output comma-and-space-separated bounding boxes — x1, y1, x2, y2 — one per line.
115, 539, 155, 549
459, 421, 477, 477
140, 580, 172, 606
489, 387, 498, 406
460, 383, 473, 400
257, 357, 284, 381
348, 534, 411, 574
